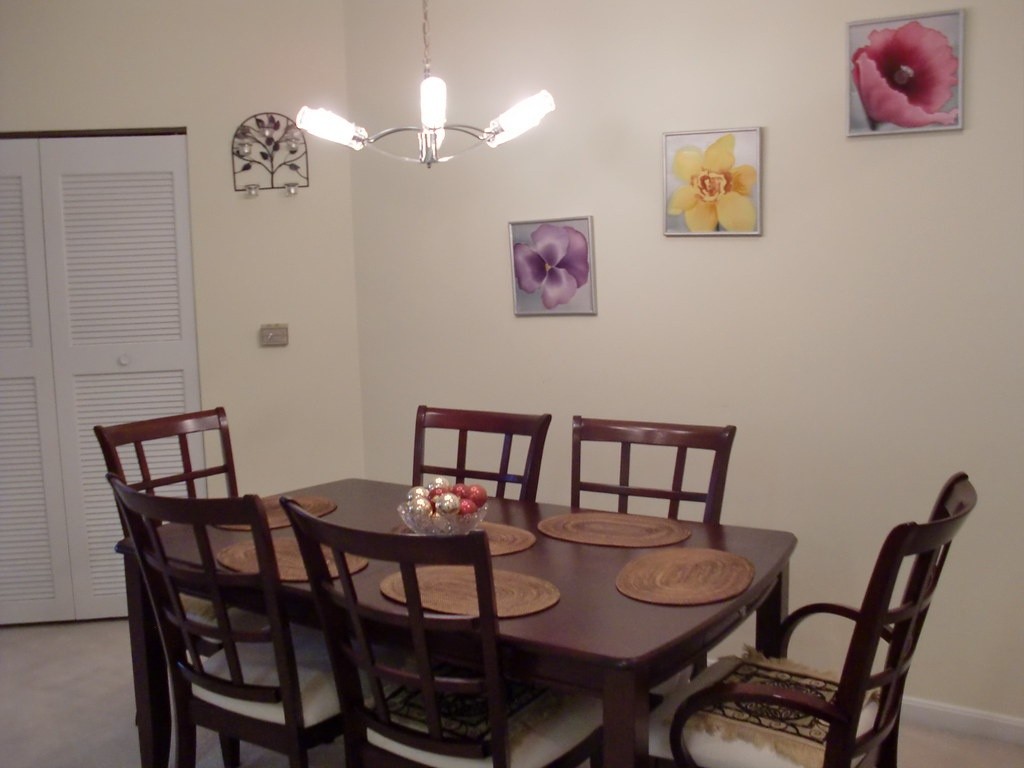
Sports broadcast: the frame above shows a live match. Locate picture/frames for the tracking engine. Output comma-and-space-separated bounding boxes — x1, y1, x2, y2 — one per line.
509, 216, 598, 319
844, 10, 966, 140
657, 126, 763, 239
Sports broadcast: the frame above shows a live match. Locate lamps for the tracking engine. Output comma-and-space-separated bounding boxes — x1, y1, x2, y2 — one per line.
295, 0, 553, 169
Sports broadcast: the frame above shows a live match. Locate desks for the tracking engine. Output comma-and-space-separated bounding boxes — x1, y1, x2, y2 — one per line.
111, 475, 797, 768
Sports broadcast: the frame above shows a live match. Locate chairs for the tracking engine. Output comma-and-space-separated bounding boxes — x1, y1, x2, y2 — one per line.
107, 469, 411, 768
565, 415, 741, 698
405, 405, 552, 503
279, 493, 610, 768
93, 407, 287, 648
633, 474, 980, 765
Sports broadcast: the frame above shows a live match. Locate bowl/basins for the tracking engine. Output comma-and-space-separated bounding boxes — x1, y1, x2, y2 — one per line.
396, 497, 489, 535
